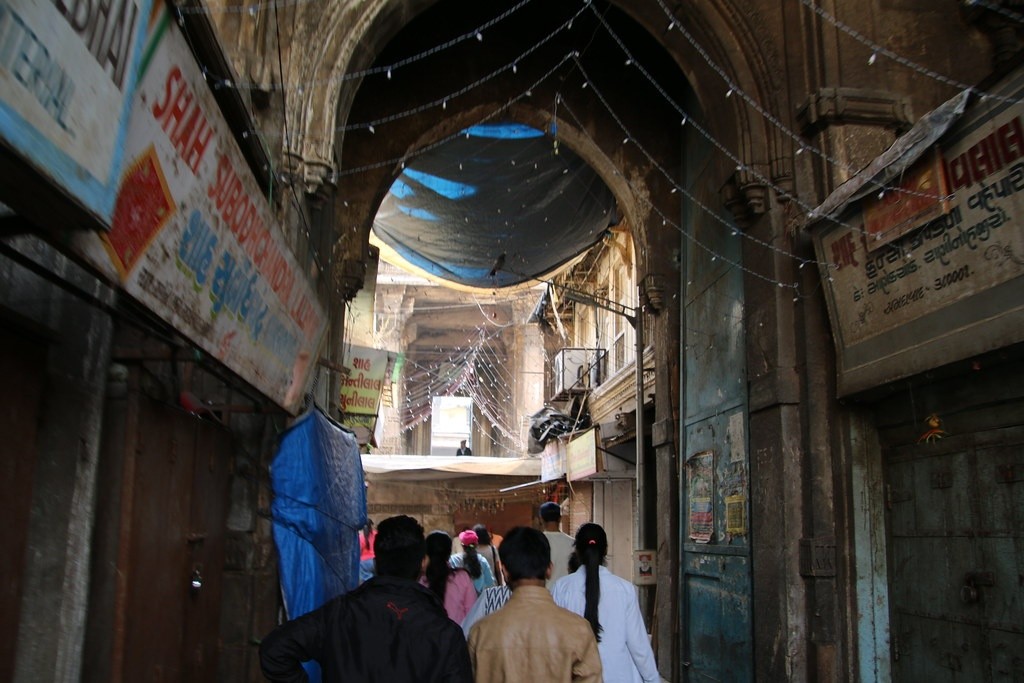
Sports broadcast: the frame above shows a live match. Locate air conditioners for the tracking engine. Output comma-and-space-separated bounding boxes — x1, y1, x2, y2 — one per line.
555, 350, 595, 394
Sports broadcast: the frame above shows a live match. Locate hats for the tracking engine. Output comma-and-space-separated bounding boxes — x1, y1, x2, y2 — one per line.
459, 530, 478, 547
539, 501, 560, 517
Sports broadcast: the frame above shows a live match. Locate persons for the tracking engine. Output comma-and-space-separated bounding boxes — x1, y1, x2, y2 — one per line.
472, 523, 506, 586
260, 515, 475, 683
356, 518, 379, 584
417, 531, 478, 626
449, 528, 498, 598
551, 523, 665, 683
538, 502, 577, 591
462, 584, 513, 641
468, 526, 604, 683
457, 440, 471, 455
